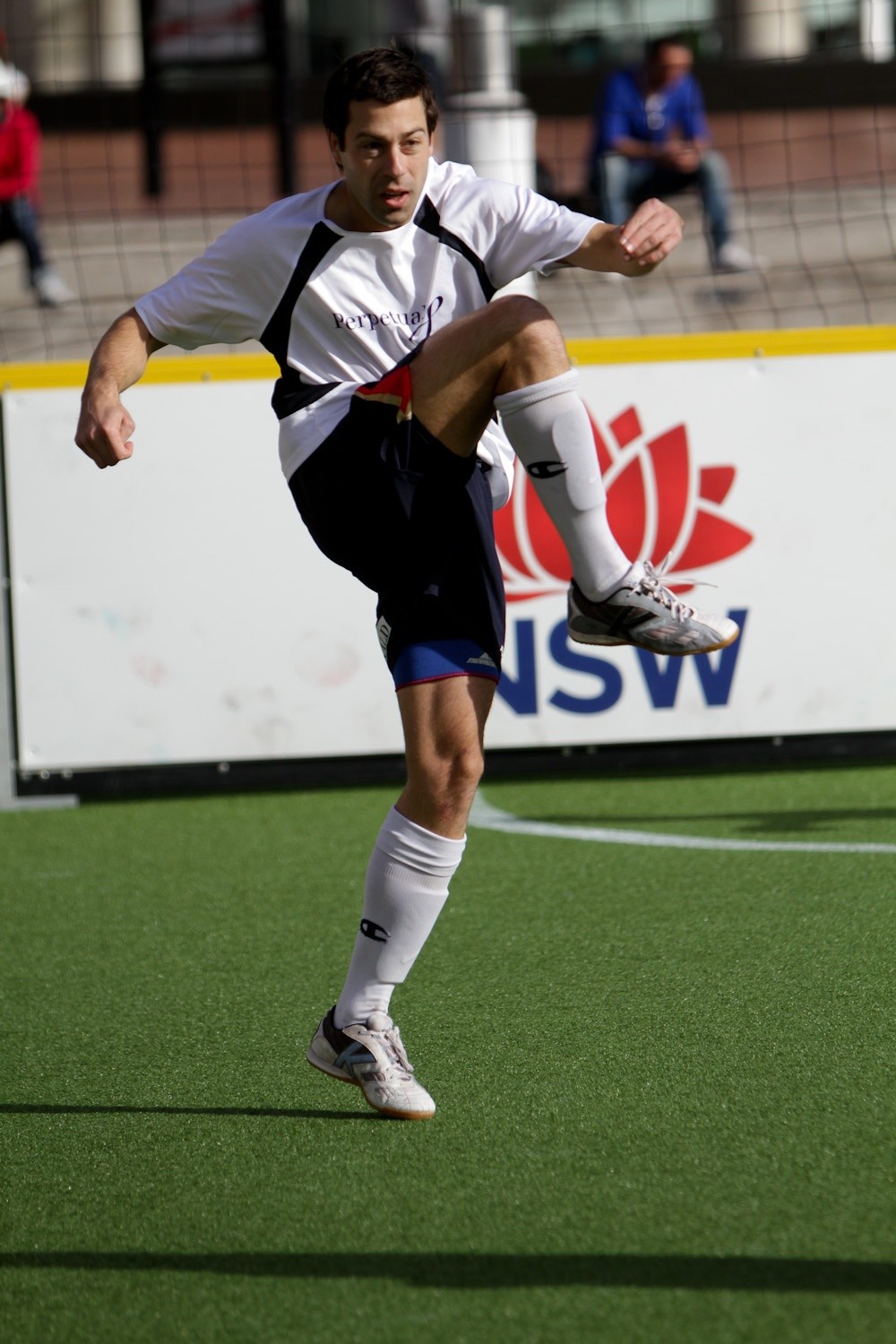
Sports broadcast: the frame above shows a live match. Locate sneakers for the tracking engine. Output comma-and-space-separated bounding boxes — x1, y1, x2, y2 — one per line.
306, 1004, 436, 1119
567, 551, 739, 656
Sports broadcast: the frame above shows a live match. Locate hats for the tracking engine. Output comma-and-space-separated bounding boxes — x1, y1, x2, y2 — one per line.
0, 60, 31, 101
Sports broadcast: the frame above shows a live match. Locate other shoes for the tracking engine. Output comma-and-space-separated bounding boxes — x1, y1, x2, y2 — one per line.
29, 275, 69, 308
712, 243, 755, 270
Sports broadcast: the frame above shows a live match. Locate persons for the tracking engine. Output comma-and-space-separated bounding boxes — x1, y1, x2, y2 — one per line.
1, 62, 78, 311
76, 50, 740, 1121
587, 34, 766, 273
